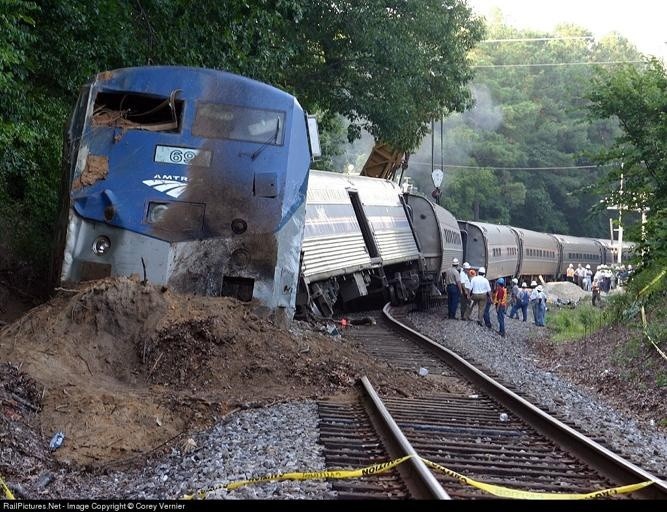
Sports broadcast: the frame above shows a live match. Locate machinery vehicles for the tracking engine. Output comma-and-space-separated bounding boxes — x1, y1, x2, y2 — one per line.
359, 59, 445, 204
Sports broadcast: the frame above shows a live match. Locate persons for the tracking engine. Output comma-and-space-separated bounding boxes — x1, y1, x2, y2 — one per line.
535, 286, 547, 326
510, 282, 530, 322
530, 281, 539, 323
459, 261, 493, 330
494, 278, 508, 336
591, 280, 602, 308
446, 257, 462, 319
504, 278, 519, 320
566, 262, 637, 291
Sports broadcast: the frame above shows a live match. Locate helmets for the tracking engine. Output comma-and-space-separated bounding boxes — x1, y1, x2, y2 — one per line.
463, 261, 471, 268
596, 264, 608, 269
452, 258, 459, 265
496, 277, 505, 285
569, 263, 591, 269
479, 266, 486, 273
511, 278, 543, 292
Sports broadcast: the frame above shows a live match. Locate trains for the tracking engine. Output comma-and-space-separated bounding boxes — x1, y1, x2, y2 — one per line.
60, 65, 648, 322
